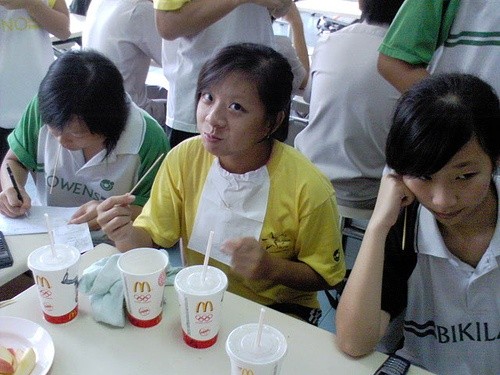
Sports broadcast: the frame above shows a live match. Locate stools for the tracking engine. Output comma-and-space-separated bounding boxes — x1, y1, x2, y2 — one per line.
329, 178, 383, 308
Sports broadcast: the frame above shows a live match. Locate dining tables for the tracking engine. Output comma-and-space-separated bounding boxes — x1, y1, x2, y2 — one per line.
0, 243, 437, 375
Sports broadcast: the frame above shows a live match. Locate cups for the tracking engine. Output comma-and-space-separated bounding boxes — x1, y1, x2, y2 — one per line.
117, 247, 169, 329
174, 264, 228, 349
224, 321, 287, 375
27, 243, 81, 325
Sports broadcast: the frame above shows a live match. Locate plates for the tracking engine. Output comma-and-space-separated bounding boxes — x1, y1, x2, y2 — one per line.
0, 315, 55, 375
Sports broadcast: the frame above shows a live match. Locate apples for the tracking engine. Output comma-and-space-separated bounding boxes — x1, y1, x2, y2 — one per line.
0, 346, 17, 375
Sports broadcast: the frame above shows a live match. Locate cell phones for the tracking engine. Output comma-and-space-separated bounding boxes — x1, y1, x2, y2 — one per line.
0, 231, 13, 269
374, 356, 411, 375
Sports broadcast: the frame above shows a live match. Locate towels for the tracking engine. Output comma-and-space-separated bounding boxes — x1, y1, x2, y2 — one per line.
79, 254, 182, 328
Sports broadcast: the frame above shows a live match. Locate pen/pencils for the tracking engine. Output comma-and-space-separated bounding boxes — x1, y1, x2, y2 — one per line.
6, 163, 29, 218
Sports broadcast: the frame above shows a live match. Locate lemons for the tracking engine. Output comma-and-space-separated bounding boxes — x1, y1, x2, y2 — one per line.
12, 347, 36, 375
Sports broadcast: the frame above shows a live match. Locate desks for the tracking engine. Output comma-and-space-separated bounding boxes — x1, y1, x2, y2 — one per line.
0, 232, 51, 289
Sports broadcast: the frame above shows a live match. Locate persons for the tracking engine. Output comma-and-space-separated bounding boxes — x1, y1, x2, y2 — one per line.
270, 0, 309, 92
0, 48, 172, 231
336, 72, 500, 375
0, 0, 71, 166
96, 43, 346, 327
293, 0, 427, 209
82, 0, 166, 123
376, 0, 500, 94
153, 0, 292, 147
